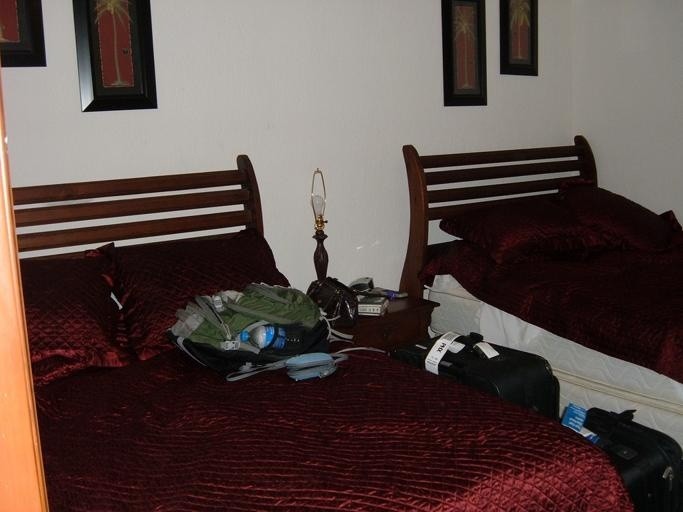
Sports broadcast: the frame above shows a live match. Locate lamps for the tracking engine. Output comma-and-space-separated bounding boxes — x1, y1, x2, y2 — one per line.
310, 168, 329, 280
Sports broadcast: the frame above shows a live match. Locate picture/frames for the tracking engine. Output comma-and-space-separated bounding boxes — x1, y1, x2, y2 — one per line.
498, 0, 539, 76
441, 0, 488, 107
72, 0, 158, 112
0, 0, 47, 68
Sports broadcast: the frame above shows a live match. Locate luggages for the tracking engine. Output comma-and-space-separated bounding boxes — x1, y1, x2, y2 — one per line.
388, 326, 561, 425
574, 408, 683, 512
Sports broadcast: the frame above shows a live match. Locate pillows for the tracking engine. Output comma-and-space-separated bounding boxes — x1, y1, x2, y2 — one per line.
113, 229, 290, 363
440, 177, 674, 265
20, 242, 131, 386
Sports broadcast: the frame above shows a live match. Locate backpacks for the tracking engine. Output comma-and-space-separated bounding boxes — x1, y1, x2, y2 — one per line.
162, 281, 333, 374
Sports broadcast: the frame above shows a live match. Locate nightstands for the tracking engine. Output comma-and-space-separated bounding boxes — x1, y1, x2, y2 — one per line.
304, 287, 440, 348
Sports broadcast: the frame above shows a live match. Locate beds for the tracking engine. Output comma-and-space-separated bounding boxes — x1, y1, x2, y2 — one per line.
12, 153, 607, 512
398, 134, 682, 449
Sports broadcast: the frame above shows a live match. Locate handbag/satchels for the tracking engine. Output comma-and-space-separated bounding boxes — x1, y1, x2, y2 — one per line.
307, 277, 362, 328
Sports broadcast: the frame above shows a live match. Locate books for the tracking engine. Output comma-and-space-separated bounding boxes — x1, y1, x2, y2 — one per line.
357, 297, 389, 316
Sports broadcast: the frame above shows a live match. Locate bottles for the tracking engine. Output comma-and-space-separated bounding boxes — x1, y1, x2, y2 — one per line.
237, 325, 284, 352
